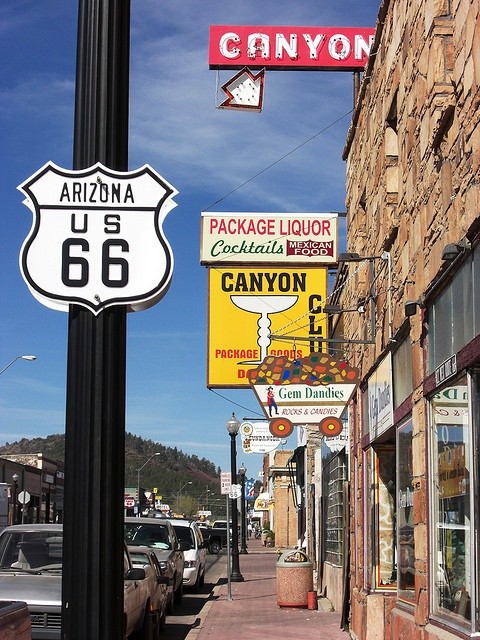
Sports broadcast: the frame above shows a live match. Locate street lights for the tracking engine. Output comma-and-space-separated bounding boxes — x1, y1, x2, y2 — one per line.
198, 490, 210, 511
209, 499, 220, 510
238, 463, 247, 554
0, 354, 36, 381
227, 412, 243, 582
138, 453, 160, 500
200, 493, 214, 508
177, 482, 192, 503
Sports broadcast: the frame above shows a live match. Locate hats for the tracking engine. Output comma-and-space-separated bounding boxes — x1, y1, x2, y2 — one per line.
268, 386, 273, 390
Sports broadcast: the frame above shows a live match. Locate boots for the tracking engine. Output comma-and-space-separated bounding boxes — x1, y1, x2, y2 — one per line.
275, 408, 279, 414
269, 409, 272, 417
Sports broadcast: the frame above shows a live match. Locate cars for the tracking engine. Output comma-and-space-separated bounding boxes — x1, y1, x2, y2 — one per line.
159, 516, 208, 594
213, 519, 234, 539
1, 524, 151, 639
123, 517, 188, 639
196, 522, 228, 555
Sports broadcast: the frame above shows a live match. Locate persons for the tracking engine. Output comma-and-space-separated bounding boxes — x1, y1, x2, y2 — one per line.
267, 386, 279, 417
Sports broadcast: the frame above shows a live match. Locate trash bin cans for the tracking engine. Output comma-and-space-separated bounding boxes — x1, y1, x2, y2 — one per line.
275, 550, 313, 607
